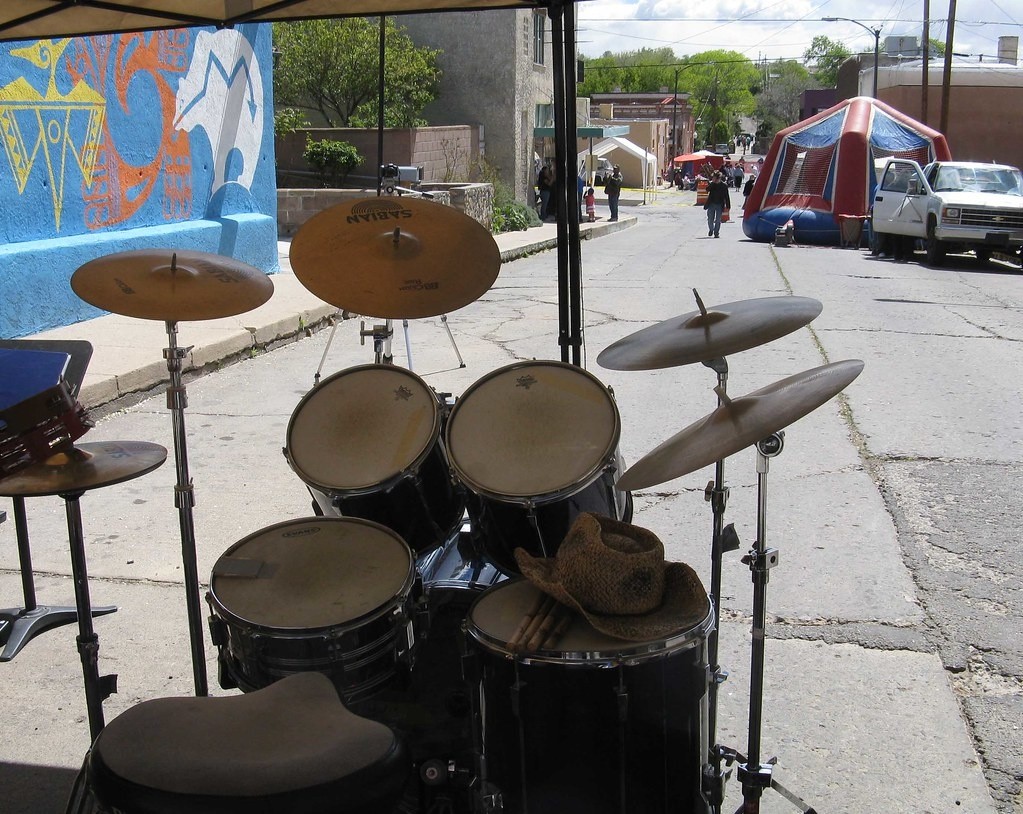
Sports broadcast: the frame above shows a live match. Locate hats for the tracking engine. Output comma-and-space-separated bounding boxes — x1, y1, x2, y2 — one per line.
613, 164, 620, 169
513, 513, 711, 640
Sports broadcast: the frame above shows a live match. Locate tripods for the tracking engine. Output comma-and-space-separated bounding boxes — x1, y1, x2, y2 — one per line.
313, 179, 466, 387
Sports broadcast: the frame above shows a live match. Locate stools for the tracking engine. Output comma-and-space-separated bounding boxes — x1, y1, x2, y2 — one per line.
82, 669, 413, 812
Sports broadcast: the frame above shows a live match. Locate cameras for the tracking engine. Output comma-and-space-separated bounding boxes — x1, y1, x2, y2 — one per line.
379, 163, 399, 177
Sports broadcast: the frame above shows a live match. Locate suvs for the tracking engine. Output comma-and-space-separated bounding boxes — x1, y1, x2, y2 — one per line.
872, 158, 1023, 270
715, 143, 729, 156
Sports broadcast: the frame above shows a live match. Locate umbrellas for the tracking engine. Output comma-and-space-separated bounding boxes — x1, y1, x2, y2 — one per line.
693, 149, 723, 157
673, 153, 705, 161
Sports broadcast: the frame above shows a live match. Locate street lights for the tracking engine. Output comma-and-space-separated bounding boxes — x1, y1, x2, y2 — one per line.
671, 61, 715, 186
821, 17, 883, 98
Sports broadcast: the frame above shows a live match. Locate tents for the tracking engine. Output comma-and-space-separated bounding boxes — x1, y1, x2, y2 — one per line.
578, 136, 658, 205
742, 96, 953, 245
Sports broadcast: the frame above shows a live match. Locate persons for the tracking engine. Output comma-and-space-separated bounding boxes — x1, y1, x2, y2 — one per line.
742, 174, 755, 210
734, 134, 751, 150
868, 170, 896, 256
602, 163, 623, 222
537, 165, 552, 219
733, 164, 744, 192
757, 156, 764, 164
725, 155, 731, 161
578, 176, 584, 222
583, 187, 596, 221
739, 157, 745, 164
887, 168, 915, 263
703, 170, 731, 238
665, 161, 759, 190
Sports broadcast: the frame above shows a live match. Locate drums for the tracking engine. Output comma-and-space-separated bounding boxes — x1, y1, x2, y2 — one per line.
210, 515, 433, 705
464, 580, 716, 813
283, 362, 466, 559
445, 357, 635, 580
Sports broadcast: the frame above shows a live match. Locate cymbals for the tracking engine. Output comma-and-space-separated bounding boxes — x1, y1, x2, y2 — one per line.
0, 440, 167, 498
595, 295, 823, 372
69, 247, 275, 321
616, 360, 866, 491
289, 195, 502, 319
